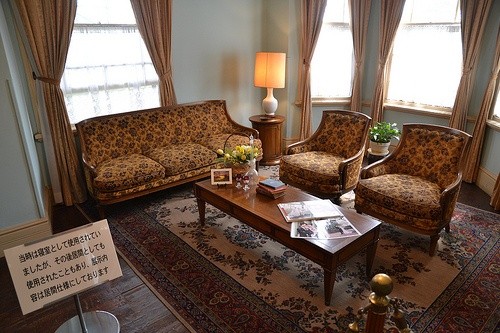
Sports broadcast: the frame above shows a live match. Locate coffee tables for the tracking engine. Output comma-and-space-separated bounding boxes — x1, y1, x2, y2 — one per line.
196, 175, 381, 308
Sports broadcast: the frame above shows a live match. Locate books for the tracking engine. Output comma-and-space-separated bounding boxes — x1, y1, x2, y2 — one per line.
277, 198, 344, 223
256, 178, 287, 199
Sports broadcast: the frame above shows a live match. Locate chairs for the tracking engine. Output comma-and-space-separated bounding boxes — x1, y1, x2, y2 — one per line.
279, 110, 373, 205
356, 123, 472, 257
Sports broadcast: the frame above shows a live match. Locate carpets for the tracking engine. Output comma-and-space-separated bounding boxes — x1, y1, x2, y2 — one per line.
74, 183, 500, 333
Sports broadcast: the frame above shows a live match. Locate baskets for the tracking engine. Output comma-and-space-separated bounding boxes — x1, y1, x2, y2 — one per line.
223, 132, 254, 175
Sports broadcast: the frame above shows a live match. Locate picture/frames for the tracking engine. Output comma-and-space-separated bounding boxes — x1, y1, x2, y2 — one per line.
211, 168, 233, 185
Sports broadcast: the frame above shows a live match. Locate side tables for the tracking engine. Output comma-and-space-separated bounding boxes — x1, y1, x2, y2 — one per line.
367, 148, 391, 165
249, 115, 286, 165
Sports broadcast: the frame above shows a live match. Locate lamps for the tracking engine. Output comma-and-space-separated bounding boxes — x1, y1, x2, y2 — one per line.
252, 51, 287, 115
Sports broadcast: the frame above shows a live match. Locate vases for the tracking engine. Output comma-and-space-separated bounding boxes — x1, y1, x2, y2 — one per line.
236, 158, 256, 174
372, 138, 390, 153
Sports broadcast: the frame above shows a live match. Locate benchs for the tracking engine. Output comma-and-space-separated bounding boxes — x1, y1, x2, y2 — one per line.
74, 99, 260, 220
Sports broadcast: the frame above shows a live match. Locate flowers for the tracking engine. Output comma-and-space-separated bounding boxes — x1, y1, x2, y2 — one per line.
217, 144, 258, 163
369, 121, 401, 143
